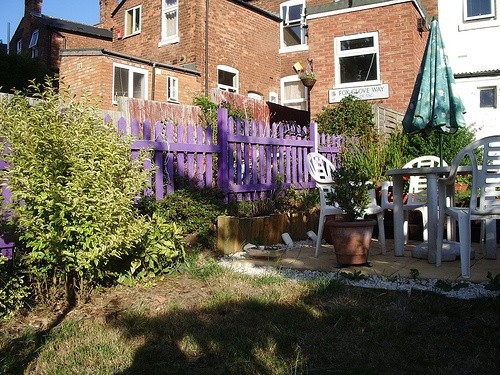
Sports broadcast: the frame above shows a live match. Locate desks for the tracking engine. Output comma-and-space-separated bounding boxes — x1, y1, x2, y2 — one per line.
384, 166, 473, 264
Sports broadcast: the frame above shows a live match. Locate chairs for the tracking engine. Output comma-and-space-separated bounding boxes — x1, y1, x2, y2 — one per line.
436, 135, 500, 278
306, 152, 386, 258
381, 155, 452, 244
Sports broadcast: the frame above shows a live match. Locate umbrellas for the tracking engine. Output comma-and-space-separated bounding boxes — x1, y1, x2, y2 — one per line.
400, 14, 467, 168
